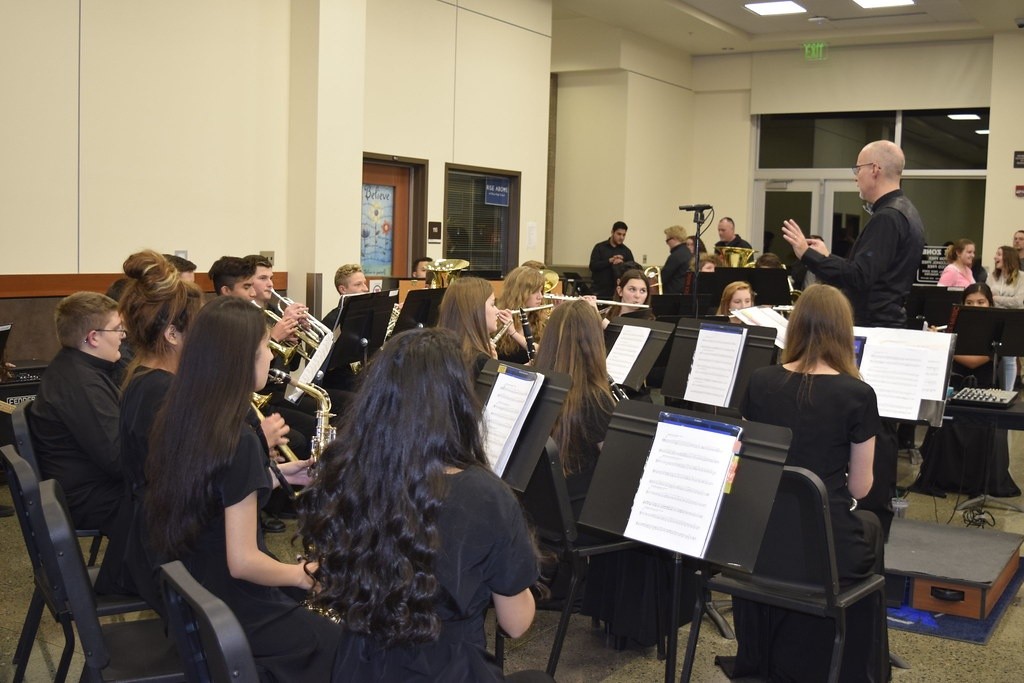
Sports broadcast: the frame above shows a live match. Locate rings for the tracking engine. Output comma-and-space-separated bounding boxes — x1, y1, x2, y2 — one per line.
794, 235, 798, 240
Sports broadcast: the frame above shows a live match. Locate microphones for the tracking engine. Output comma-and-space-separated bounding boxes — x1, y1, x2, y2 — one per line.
679, 204, 713, 211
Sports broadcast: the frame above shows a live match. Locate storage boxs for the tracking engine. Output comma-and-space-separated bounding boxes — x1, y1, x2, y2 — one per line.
884, 573, 908, 609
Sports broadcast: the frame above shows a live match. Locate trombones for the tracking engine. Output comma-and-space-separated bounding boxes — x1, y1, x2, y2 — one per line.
644, 266, 663, 295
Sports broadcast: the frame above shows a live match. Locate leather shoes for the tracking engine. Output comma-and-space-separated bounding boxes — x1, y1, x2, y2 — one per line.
261, 512, 285, 532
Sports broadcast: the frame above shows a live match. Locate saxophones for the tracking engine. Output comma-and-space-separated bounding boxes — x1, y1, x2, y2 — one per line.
268, 367, 338, 480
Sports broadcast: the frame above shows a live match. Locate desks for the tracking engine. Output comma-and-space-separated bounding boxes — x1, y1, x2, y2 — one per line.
944, 398, 1024, 503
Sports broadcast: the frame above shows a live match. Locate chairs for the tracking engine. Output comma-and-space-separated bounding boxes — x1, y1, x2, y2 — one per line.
159, 559, 262, 683
0, 444, 151, 683
681, 465, 891, 683
11, 399, 103, 568
39, 478, 186, 683
516, 435, 666, 679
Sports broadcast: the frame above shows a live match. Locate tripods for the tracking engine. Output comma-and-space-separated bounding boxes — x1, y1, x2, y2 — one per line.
950, 306, 1024, 512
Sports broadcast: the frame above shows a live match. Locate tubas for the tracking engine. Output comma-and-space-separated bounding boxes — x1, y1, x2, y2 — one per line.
424, 259, 470, 288
539, 268, 560, 317
713, 245, 759, 268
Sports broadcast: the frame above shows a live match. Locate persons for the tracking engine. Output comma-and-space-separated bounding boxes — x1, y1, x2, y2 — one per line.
291, 328, 549, 683
437, 260, 613, 368
532, 299, 710, 648
911, 282, 1022, 498
35, 250, 315, 531
783, 140, 926, 513
714, 285, 892, 683
660, 217, 824, 325
936, 230, 1024, 391
412, 257, 432, 279
590, 221, 656, 322
140, 297, 338, 683
321, 263, 368, 334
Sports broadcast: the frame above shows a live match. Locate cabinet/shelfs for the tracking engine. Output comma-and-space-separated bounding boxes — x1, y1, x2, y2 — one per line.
909, 541, 1020, 621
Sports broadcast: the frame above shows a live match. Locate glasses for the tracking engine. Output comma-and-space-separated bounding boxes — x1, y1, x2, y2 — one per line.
851, 163, 881, 175
84, 323, 127, 343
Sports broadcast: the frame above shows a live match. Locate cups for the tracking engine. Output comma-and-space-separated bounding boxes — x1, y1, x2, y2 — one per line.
892, 497, 909, 519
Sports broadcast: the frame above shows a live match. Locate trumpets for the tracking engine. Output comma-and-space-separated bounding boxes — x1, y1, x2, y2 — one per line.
250, 298, 319, 361
272, 289, 332, 343
268, 338, 299, 367
252, 390, 316, 499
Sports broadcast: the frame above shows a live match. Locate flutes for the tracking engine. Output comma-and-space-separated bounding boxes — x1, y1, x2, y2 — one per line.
512, 304, 554, 313
492, 320, 512, 343
544, 293, 649, 308
520, 307, 536, 365
